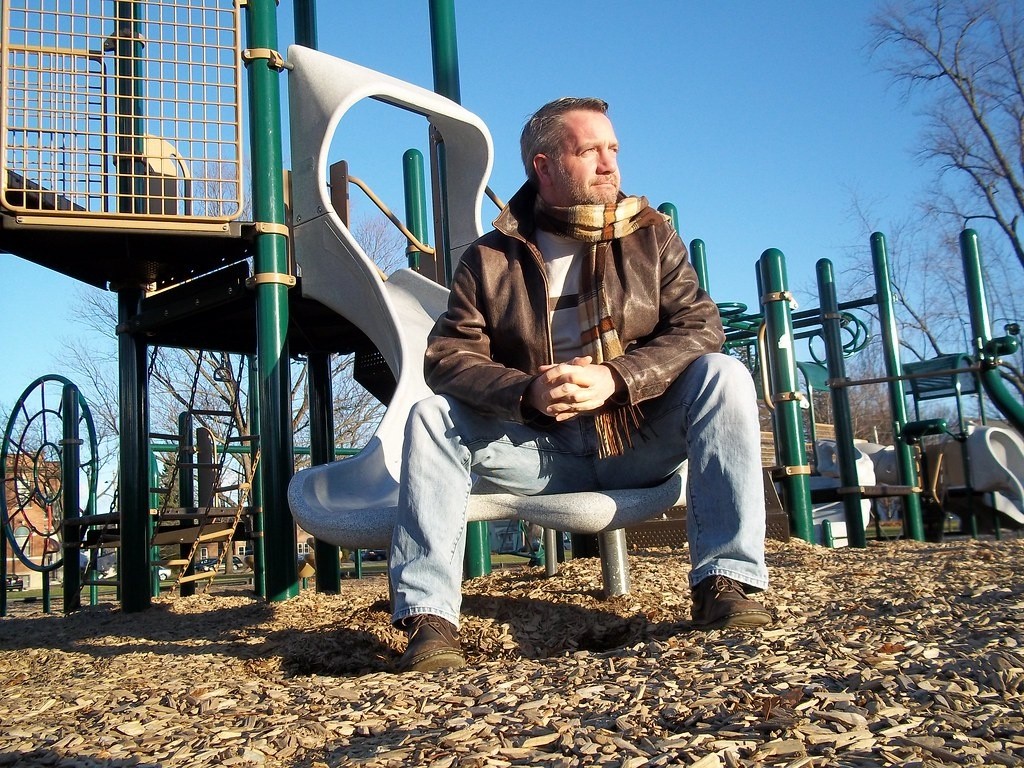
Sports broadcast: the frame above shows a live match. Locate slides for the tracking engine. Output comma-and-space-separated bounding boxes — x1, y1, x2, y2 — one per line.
289, 44, 690, 551
947, 425, 1024, 527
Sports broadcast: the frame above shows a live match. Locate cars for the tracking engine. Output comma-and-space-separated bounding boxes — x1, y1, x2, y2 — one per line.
519, 543, 544, 553
194, 558, 218, 573
350, 549, 387, 562
159, 566, 172, 581
564, 540, 572, 550
6, 574, 23, 591
203, 556, 244, 571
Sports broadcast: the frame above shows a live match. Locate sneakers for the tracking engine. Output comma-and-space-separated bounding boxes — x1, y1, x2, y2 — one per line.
691, 574, 772, 629
399, 613, 465, 672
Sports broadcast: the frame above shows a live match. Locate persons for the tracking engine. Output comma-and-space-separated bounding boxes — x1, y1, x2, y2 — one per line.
385, 98, 773, 671
